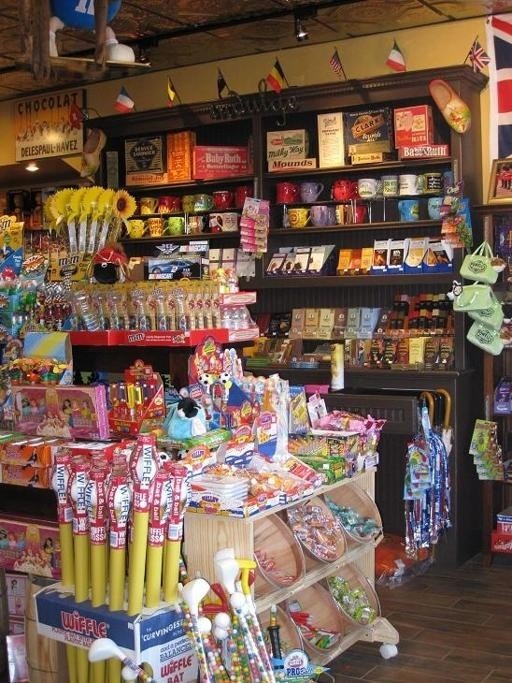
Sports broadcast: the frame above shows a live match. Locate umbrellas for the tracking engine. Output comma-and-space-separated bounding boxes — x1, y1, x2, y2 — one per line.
422, 389, 454, 457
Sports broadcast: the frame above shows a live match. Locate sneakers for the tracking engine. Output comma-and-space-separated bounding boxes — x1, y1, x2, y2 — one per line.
428, 79, 472, 136
79, 127, 108, 179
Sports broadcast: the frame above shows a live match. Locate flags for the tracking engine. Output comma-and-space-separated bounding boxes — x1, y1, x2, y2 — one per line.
167, 79, 176, 109
468, 39, 491, 74
113, 88, 135, 114
485, 14, 512, 158
217, 69, 226, 99
385, 40, 406, 73
266, 62, 283, 94
330, 52, 342, 77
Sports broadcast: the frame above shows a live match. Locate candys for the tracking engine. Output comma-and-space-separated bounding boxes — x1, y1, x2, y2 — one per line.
123, 657, 156, 683
178, 555, 275, 683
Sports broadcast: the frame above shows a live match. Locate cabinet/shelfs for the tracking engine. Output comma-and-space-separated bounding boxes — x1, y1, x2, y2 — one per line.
0, 62, 512, 568
183, 438, 383, 682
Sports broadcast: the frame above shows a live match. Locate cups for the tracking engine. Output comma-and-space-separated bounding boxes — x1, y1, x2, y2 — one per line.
125, 188, 250, 238
278, 173, 452, 229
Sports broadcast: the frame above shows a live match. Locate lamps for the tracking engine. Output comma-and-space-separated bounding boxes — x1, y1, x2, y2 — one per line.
139, 44, 148, 60
296, 17, 309, 38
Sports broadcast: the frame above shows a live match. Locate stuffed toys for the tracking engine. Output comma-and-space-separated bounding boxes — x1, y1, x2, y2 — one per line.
162, 395, 210, 440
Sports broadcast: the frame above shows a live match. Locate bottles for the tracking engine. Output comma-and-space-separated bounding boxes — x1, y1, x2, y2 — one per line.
387, 292, 452, 328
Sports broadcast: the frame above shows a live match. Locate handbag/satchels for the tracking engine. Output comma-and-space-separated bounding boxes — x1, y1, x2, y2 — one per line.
452, 242, 505, 356
